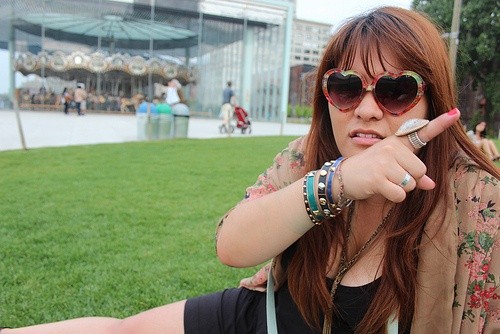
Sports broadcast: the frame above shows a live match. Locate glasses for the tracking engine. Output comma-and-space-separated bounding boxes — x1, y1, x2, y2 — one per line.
322, 68, 428, 118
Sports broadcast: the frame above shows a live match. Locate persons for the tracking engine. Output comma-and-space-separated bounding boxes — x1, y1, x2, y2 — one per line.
466, 118, 500, 161
0, 7, 500, 334
26, 76, 238, 136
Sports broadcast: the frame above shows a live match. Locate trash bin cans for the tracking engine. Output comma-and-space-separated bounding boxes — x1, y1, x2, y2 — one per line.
135, 102, 190, 140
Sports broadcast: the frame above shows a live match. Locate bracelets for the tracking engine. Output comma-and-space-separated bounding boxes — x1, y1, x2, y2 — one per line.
304, 156, 353, 226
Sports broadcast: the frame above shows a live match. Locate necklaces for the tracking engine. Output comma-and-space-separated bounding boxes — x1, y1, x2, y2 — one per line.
323, 198, 398, 334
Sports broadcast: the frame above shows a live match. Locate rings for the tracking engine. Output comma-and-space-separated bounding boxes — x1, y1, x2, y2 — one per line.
399, 172, 412, 187
395, 117, 429, 151
